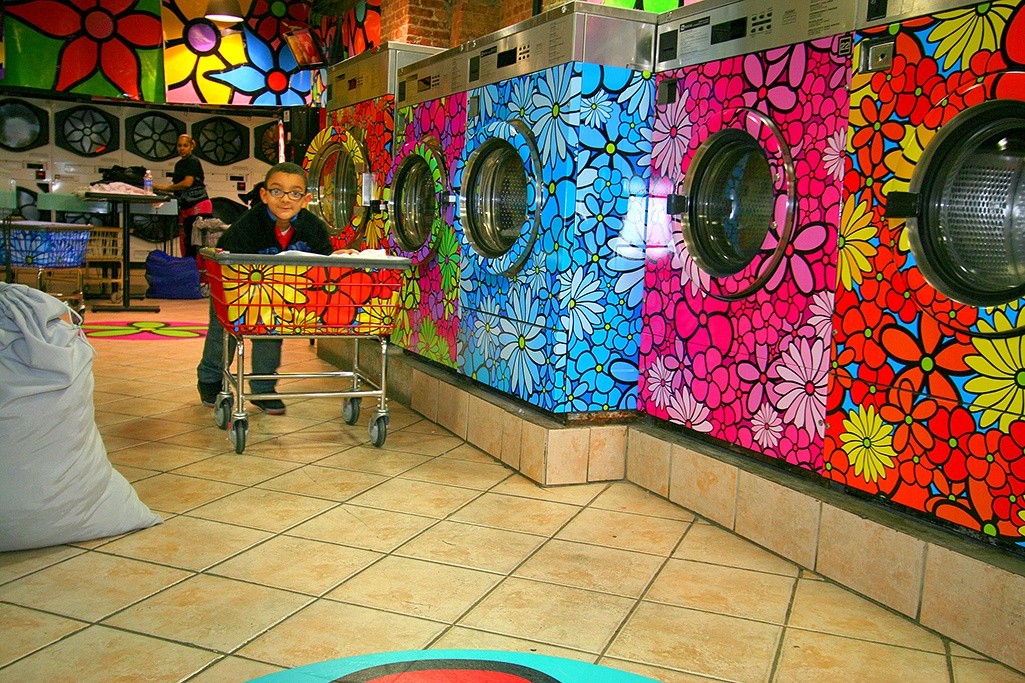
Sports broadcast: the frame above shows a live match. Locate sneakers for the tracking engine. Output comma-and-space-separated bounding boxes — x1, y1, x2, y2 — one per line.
197, 379, 221, 406
250, 391, 285, 415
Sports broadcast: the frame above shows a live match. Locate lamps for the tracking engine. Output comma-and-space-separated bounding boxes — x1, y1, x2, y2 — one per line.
204, 0, 245, 22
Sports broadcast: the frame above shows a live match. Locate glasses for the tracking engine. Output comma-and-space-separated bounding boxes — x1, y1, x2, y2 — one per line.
265, 187, 306, 201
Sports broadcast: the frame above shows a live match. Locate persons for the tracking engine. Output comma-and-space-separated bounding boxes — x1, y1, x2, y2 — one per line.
152, 134, 213, 258
197, 163, 334, 415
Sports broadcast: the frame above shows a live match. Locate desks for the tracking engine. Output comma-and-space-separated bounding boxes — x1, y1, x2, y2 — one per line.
36, 191, 171, 311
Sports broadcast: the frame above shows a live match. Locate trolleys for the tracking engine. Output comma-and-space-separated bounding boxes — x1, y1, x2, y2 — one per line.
0, 213, 124, 316
201, 247, 412, 454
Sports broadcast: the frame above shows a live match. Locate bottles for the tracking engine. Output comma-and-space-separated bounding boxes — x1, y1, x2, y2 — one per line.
144, 170, 153, 192
